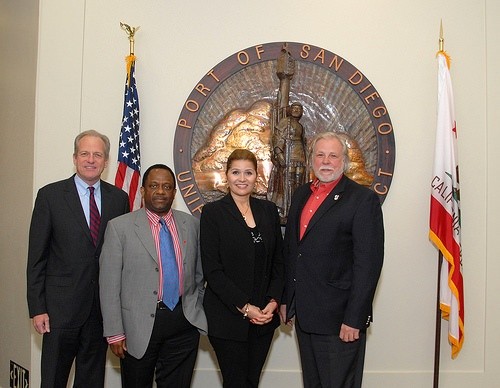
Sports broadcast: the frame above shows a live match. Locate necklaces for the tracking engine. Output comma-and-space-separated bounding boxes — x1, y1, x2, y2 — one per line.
240, 208, 249, 221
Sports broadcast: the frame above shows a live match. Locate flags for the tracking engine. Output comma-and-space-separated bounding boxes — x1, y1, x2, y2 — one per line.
428, 49, 466, 359
115, 55, 143, 213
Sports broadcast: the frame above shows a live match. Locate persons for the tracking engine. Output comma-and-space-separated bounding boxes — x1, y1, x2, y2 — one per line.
200, 149, 284, 388
98, 164, 208, 388
279, 131, 384, 388
272, 102, 305, 216
26, 130, 130, 388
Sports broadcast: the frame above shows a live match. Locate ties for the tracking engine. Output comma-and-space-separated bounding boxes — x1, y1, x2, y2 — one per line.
159, 218, 179, 311
87, 187, 101, 247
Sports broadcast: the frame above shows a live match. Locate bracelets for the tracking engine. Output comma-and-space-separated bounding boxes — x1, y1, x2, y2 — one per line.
244, 304, 250, 316
